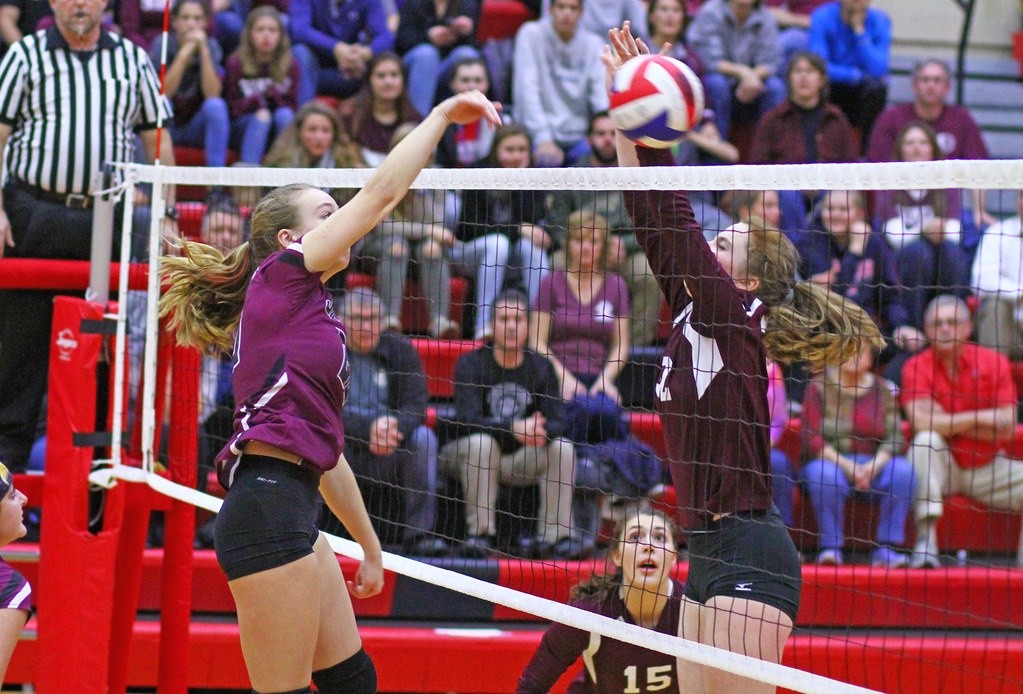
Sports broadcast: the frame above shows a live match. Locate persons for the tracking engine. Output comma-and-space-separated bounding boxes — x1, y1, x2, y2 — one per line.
440, 289, 597, 560
0, 0, 1023, 694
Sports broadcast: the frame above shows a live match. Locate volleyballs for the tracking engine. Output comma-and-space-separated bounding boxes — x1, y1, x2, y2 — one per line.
610, 54, 705, 150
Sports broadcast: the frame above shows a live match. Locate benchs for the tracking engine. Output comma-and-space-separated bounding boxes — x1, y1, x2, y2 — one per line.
0, 0, 1023, 694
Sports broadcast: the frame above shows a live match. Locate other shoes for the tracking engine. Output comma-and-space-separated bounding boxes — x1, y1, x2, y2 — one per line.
462, 535, 493, 557
910, 552, 941, 568
872, 547, 909, 568
815, 549, 844, 566
541, 537, 593, 561
404, 535, 448, 558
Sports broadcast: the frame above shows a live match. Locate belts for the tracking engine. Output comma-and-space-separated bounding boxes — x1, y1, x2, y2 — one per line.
10, 177, 93, 209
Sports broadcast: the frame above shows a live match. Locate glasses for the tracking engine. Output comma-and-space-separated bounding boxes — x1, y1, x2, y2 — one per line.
340, 312, 384, 325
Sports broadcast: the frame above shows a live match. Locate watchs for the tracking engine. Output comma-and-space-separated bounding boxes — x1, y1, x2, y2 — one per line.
166, 207, 179, 220
